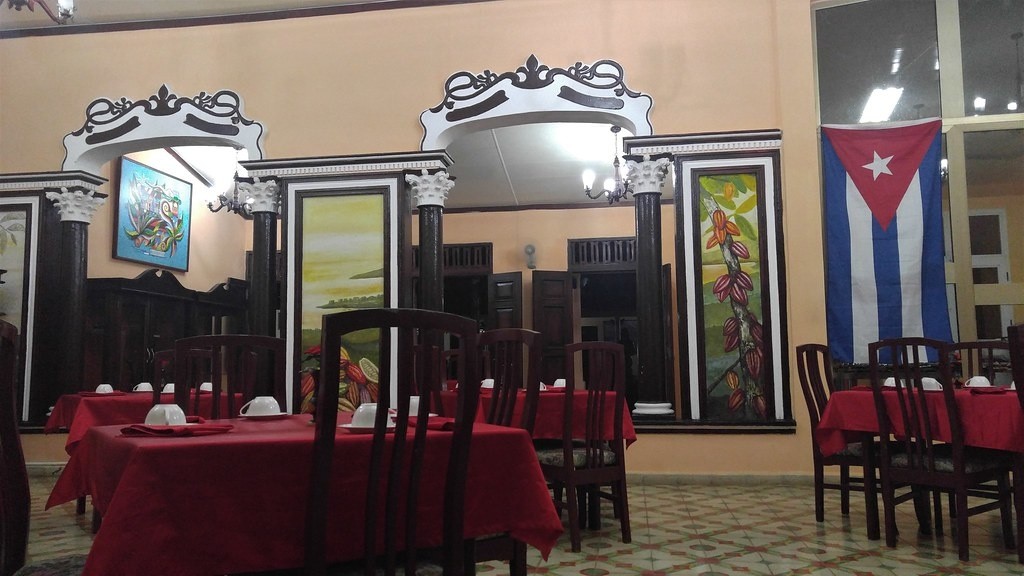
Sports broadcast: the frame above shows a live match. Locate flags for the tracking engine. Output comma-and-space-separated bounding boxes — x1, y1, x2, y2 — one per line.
821, 117, 955, 363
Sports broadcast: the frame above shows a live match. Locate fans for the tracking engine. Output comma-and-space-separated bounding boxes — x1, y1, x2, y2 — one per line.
514, 237, 545, 269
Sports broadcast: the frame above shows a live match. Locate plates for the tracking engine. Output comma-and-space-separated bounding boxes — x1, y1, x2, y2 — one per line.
922, 389, 943, 392
132, 390, 154, 393
161, 392, 174, 394
965, 386, 996, 387
390, 413, 438, 418
1004, 388, 1017, 390
522, 389, 549, 391
338, 423, 396, 434
238, 412, 287, 420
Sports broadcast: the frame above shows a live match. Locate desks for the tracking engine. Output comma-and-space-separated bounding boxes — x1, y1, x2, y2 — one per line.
430, 385, 637, 529
45, 392, 242, 535
46, 407, 564, 576
813, 383, 1024, 564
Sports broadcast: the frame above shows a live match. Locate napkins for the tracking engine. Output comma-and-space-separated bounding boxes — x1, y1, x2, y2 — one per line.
80, 392, 127, 396
454, 388, 484, 394
548, 387, 566, 393
850, 384, 905, 392
970, 387, 1006, 395
120, 425, 234, 436
186, 415, 205, 424
405, 416, 455, 431
196, 390, 211, 394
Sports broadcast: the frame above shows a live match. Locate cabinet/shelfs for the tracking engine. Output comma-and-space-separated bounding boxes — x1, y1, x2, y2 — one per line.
81, 270, 229, 392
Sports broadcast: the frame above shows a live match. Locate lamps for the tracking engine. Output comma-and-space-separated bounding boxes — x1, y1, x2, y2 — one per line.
940, 158, 949, 182
974, 32, 1024, 115
206, 146, 242, 214
0, 0, 76, 25
581, 126, 634, 205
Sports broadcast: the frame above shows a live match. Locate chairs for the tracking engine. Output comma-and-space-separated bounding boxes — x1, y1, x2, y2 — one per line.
795, 323, 1024, 565
0, 307, 633, 576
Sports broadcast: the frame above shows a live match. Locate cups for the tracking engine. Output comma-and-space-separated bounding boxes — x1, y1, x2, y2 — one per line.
408, 395, 420, 416
163, 383, 174, 392
240, 396, 280, 414
554, 379, 566, 387
351, 403, 397, 427
883, 377, 902, 387
964, 375, 990, 387
145, 404, 186, 426
540, 382, 546, 390
1011, 381, 1015, 389
132, 382, 152, 391
200, 383, 212, 392
481, 379, 494, 388
95, 384, 113, 393
921, 377, 943, 389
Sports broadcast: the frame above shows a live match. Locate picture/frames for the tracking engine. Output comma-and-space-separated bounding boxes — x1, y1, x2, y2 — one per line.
0, 203, 33, 421
112, 156, 193, 272
292, 185, 390, 413
690, 164, 776, 424
